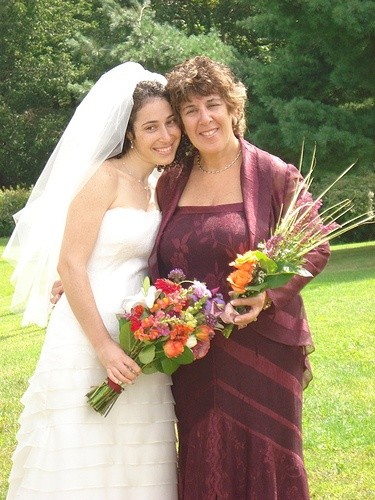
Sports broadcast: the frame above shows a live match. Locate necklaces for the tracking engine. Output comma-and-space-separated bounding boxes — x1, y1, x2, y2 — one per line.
197, 150, 242, 174
120, 157, 150, 190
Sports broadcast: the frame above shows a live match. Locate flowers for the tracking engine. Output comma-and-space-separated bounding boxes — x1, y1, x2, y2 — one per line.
85, 267, 225, 418
221, 137, 375, 339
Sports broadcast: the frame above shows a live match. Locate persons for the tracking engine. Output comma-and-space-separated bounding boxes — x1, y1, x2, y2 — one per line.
2, 60, 178, 500
51, 56, 330, 500
